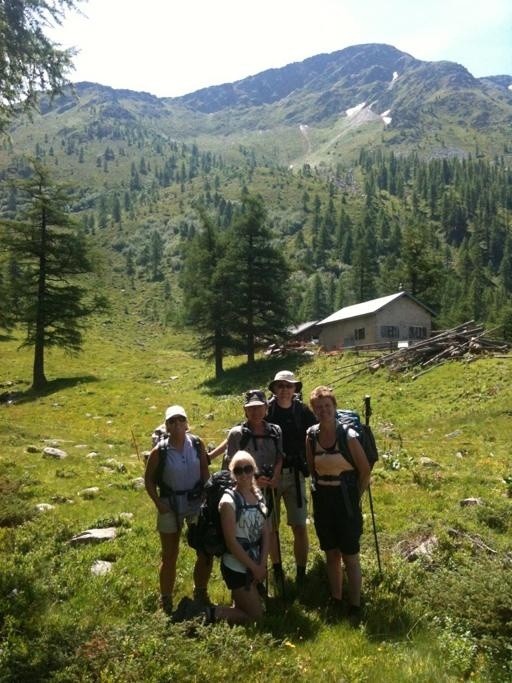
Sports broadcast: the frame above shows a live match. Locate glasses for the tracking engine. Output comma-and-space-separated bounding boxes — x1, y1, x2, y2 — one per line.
277, 384, 294, 389
167, 416, 186, 424
233, 464, 254, 475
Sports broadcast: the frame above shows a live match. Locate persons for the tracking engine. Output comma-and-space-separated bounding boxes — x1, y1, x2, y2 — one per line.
145, 405, 214, 614
227, 388, 286, 582
206, 369, 314, 586
204, 451, 272, 623
302, 385, 371, 617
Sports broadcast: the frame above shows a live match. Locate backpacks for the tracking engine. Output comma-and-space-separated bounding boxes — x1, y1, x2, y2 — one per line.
152, 424, 201, 499
267, 392, 302, 433
309, 409, 378, 470
186, 471, 242, 557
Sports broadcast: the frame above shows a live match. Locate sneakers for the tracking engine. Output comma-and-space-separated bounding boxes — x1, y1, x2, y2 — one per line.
158, 596, 212, 622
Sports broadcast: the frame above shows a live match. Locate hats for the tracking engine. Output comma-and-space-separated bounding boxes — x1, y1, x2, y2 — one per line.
244, 389, 268, 408
269, 370, 302, 392
166, 406, 187, 419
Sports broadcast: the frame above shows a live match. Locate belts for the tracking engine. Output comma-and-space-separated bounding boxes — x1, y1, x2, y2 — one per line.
281, 466, 302, 474
